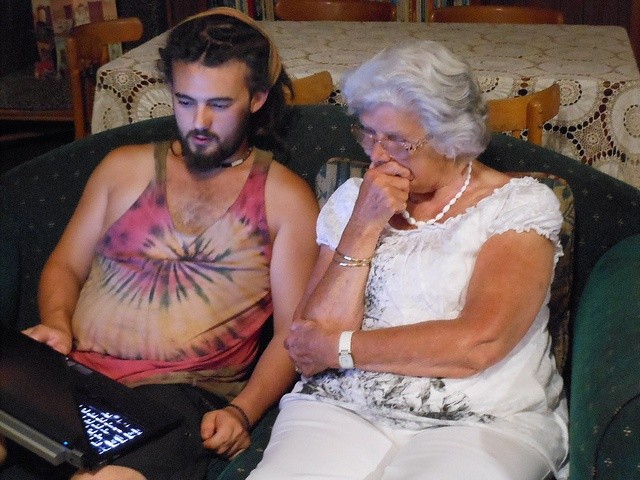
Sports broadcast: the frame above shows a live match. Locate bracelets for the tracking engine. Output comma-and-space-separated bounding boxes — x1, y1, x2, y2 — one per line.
331, 249, 376, 262
331, 262, 373, 268
225, 403, 250, 432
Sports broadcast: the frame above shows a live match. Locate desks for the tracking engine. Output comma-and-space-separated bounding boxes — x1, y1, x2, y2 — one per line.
91, 20, 640, 189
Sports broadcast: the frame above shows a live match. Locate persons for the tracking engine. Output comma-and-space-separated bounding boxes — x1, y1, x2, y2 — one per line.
248, 38, 570, 479
19, 14, 320, 480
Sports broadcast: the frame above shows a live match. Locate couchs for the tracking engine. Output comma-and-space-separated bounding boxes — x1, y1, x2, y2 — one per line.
0, 104, 640, 479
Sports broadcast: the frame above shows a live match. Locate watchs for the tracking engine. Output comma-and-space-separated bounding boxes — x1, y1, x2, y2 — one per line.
339, 330, 354, 371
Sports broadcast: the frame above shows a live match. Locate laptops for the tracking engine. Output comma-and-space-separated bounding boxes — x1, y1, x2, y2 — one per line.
0, 317, 185, 474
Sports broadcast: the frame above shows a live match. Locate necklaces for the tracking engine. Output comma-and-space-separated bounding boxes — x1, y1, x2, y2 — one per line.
168, 141, 254, 168
397, 160, 474, 225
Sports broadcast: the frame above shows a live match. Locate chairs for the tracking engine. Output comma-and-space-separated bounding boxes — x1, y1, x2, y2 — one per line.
275, 0, 396, 22
485, 82, 561, 147
66, 17, 143, 140
429, 4, 564, 24
284, 70, 333, 105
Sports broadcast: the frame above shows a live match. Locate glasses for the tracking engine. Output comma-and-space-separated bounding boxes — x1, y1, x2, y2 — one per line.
353, 126, 433, 160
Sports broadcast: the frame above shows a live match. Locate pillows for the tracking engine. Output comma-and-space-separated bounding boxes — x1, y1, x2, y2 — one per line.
314, 157, 575, 375
